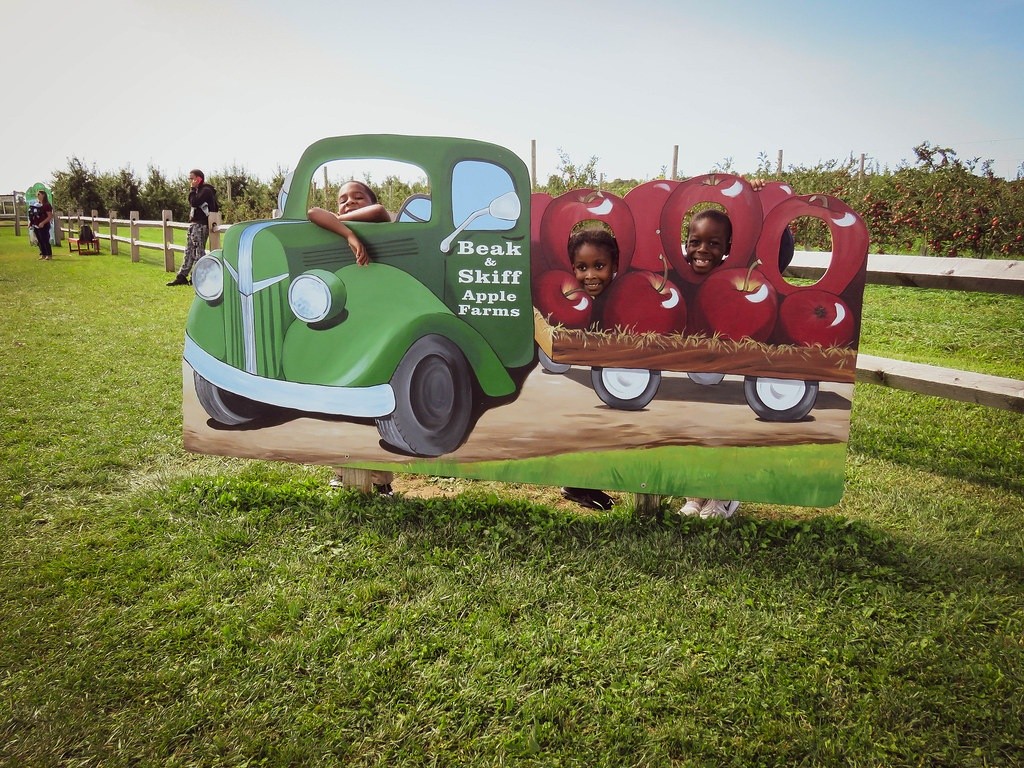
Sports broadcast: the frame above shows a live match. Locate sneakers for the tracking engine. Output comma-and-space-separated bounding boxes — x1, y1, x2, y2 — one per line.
560, 487, 615, 509
678, 497, 739, 519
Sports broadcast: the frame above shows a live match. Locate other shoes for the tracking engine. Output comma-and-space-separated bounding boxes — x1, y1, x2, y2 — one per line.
166, 274, 192, 286
373, 483, 393, 498
38, 255, 52, 260
329, 474, 343, 488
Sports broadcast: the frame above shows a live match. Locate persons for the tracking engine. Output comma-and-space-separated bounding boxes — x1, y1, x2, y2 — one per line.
165, 169, 218, 286
27, 190, 53, 261
308, 180, 399, 500
675, 209, 742, 525
561, 230, 618, 512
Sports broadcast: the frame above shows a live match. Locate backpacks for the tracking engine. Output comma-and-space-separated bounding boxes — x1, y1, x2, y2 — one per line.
79, 225, 93, 241
202, 187, 222, 212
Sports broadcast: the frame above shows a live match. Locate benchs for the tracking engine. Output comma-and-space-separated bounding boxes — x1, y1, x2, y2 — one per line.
69, 239, 99, 255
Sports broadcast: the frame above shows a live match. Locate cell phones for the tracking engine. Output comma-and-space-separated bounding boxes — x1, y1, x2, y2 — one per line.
196, 176, 202, 184
